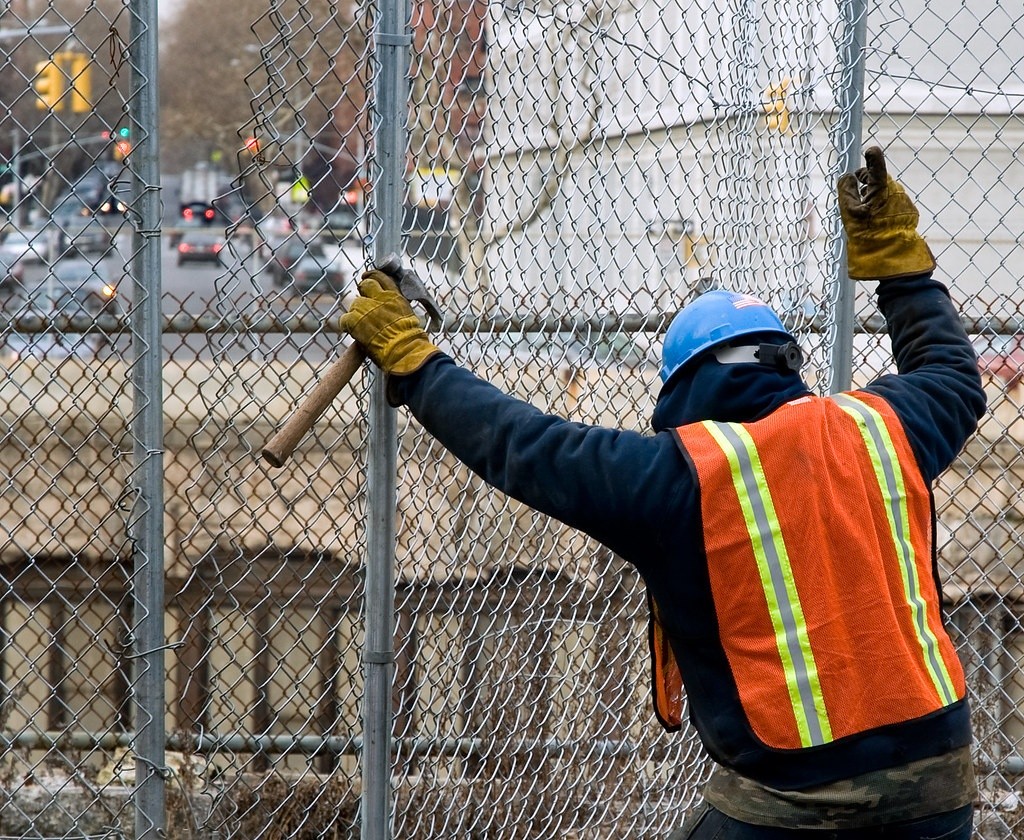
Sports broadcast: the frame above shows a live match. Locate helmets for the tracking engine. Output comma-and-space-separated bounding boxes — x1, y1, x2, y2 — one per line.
657, 289, 801, 398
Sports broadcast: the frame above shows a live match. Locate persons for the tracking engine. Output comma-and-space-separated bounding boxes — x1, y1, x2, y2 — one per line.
339, 146, 988, 840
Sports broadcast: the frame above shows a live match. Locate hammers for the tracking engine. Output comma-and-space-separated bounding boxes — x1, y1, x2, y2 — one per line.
261, 252, 444, 470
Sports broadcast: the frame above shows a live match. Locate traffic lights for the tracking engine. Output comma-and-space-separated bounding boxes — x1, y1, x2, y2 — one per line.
764, 78, 791, 135
35, 55, 64, 111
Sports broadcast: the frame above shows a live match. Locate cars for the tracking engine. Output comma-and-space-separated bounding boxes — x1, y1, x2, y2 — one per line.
57, 161, 134, 216
169, 202, 216, 249
61, 215, 113, 260
257, 211, 355, 300
176, 229, 222, 268
0, 229, 116, 362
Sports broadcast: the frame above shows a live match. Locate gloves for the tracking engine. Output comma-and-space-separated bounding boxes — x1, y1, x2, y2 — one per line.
338, 269, 443, 408
837, 146, 938, 280
